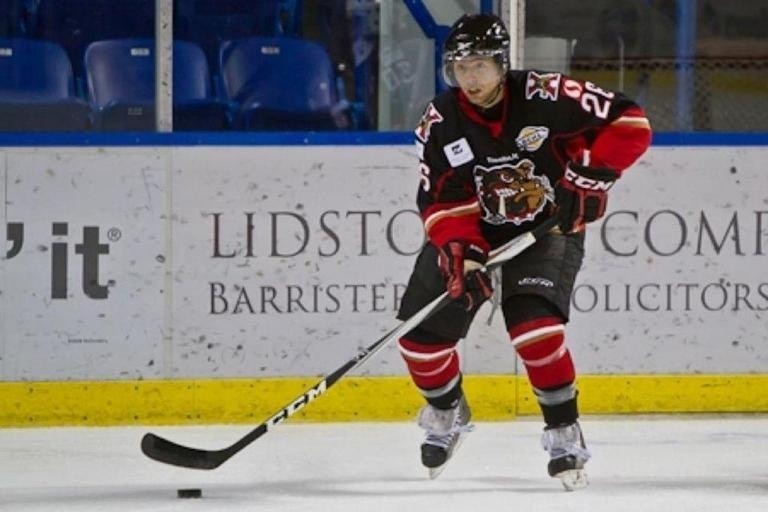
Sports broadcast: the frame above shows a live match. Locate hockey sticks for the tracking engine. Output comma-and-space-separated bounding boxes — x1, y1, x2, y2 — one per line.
141, 204, 572, 470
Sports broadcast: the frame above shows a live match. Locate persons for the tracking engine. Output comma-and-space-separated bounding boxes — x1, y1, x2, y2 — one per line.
394, 13, 653, 477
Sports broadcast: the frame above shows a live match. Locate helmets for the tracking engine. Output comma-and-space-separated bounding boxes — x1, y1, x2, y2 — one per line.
441, 12, 511, 88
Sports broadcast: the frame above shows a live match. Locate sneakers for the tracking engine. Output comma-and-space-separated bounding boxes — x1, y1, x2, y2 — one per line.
542, 418, 591, 475
416, 397, 472, 468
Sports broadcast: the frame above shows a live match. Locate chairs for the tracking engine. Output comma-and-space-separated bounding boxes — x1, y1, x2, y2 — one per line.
0, 0, 350, 132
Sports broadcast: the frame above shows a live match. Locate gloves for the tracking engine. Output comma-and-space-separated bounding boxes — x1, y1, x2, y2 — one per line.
438, 242, 494, 313
553, 160, 617, 233
